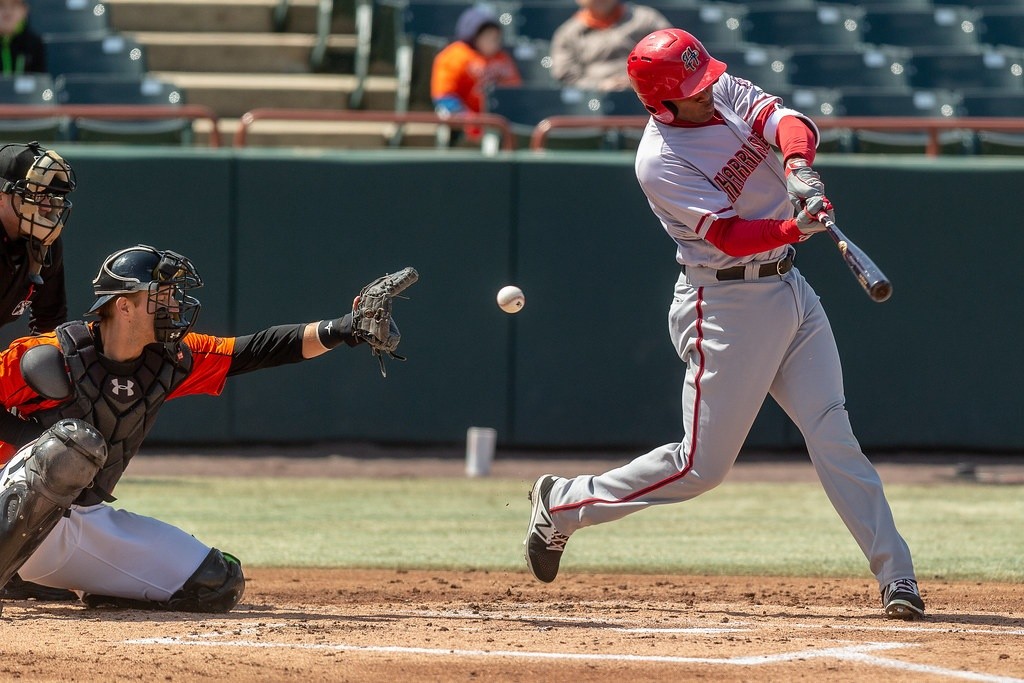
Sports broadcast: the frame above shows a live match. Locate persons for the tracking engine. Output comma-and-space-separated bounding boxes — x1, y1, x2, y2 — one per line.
0, 2, 47, 75
0, 139, 78, 338
524, 29, 928, 619
0, 244, 417, 615
547, 0, 679, 143
431, 8, 524, 143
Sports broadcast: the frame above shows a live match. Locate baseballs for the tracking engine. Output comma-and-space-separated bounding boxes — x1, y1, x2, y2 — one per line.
497, 285, 527, 315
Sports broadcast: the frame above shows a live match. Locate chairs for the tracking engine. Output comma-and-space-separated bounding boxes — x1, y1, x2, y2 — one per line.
274, 0, 1024, 154
0, 0, 197, 145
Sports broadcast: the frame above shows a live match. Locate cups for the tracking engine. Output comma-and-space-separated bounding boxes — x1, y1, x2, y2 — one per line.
465, 428, 497, 479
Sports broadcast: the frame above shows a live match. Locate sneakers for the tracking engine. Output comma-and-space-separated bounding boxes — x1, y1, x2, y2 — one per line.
880, 579, 925, 619
523, 473, 569, 584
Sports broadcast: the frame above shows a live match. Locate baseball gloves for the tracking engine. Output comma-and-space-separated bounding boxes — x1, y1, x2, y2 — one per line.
351, 265, 420, 356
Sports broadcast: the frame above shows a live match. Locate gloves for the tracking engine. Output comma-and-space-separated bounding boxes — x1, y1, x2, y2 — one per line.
796, 194, 835, 234
785, 159, 825, 212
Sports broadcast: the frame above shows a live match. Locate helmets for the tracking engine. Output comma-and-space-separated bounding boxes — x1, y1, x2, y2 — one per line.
0, 141, 71, 197
627, 28, 727, 124
82, 244, 185, 317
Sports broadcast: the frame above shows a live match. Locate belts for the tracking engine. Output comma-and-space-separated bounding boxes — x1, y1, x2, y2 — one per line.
680, 244, 796, 281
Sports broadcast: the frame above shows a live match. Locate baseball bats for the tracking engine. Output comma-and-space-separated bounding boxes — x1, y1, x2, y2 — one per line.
816, 211, 894, 304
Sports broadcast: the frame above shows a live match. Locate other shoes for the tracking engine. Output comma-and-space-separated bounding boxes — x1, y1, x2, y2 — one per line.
1, 573, 79, 602
82, 592, 129, 608
